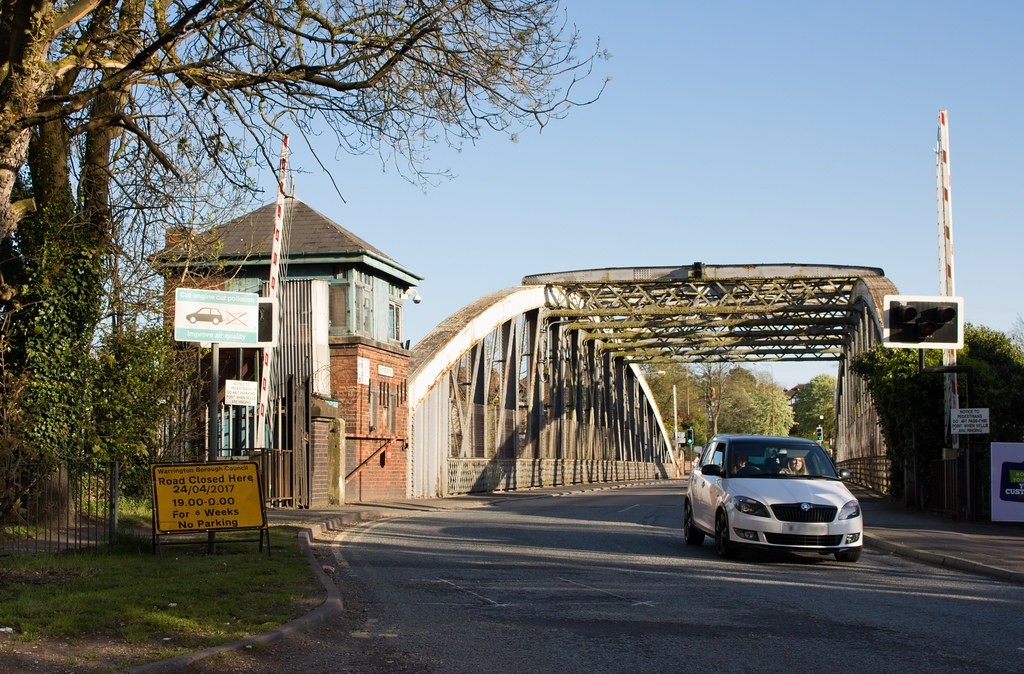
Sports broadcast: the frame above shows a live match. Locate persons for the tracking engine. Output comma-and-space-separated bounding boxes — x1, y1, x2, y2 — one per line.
720, 456, 748, 474
778, 456, 809, 475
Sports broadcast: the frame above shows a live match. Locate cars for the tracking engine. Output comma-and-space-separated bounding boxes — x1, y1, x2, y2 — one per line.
684, 432, 865, 561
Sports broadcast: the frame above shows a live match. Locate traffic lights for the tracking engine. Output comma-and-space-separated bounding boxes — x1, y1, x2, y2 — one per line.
686, 429, 693, 445
816, 427, 823, 441
880, 295, 963, 350
